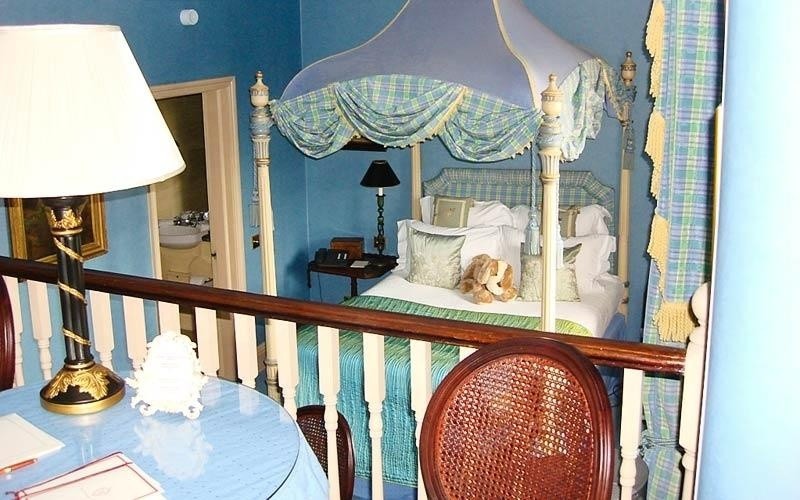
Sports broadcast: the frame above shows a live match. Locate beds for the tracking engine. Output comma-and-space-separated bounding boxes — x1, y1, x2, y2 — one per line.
281, 166, 629, 500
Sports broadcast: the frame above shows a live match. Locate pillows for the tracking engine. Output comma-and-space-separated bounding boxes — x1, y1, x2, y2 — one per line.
407, 227, 465, 289
433, 196, 472, 227
394, 221, 521, 294
511, 202, 610, 237
420, 196, 513, 226
560, 207, 579, 237
518, 242, 581, 303
517, 232, 617, 295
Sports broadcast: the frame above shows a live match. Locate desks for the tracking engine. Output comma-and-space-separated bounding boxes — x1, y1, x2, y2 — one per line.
311, 254, 398, 297
0, 373, 330, 500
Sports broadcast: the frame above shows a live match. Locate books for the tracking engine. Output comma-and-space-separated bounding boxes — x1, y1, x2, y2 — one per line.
0, 412, 67, 473
16, 450, 166, 500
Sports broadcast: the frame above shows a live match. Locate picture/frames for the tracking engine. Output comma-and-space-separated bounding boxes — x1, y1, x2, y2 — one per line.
5, 192, 108, 280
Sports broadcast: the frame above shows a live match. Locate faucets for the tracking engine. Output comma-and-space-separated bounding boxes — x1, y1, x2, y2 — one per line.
173, 213, 196, 226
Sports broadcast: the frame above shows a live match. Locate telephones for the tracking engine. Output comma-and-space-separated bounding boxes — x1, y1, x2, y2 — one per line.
314, 248, 350, 267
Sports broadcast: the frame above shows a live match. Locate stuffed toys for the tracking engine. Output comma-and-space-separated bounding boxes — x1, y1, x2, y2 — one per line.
460, 254, 518, 306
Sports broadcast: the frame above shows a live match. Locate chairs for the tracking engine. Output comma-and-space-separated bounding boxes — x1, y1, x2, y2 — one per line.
294, 404, 356, 500
419, 338, 616, 500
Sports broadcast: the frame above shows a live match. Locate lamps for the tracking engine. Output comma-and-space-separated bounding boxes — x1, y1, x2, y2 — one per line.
360, 160, 400, 256
0, 24, 186, 417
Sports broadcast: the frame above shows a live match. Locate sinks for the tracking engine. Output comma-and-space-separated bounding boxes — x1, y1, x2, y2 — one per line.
158, 219, 209, 249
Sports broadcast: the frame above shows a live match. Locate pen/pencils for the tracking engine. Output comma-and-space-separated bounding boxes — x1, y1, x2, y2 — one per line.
0, 458, 37, 474
